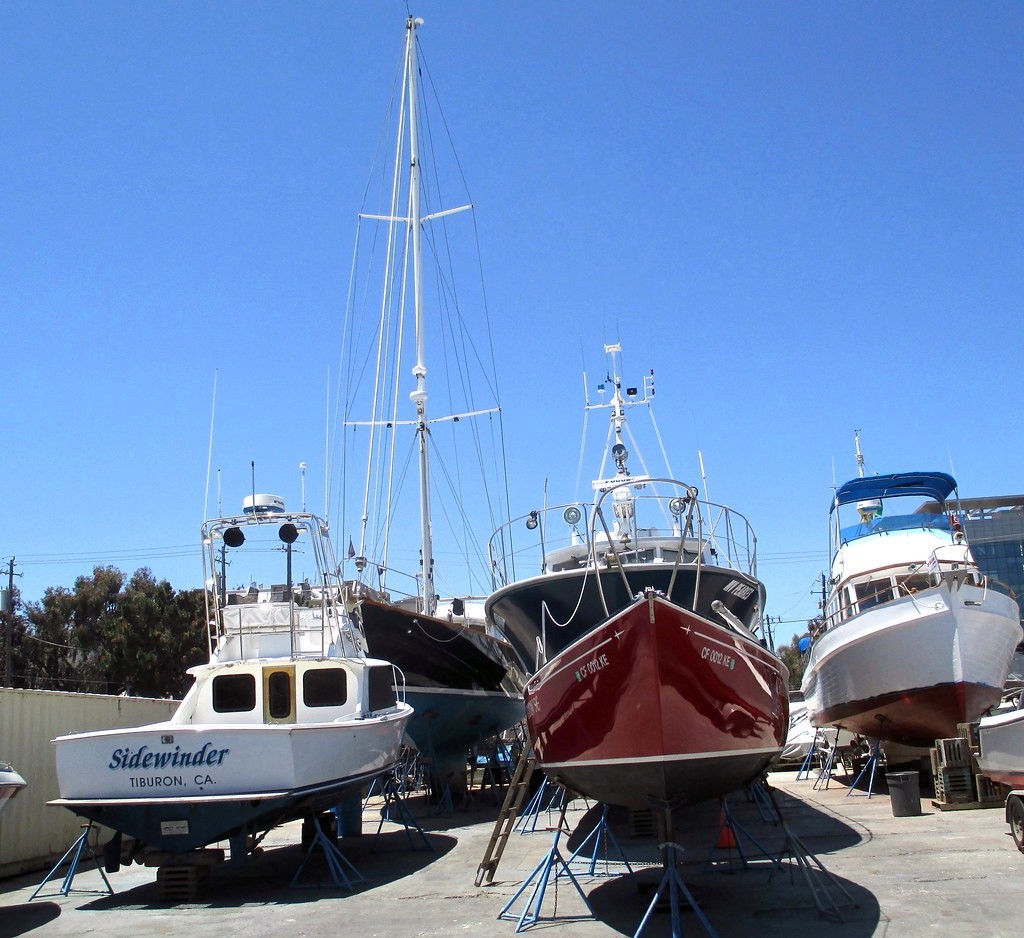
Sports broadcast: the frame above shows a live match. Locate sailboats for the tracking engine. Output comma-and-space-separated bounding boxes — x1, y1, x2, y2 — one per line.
201, 9, 525, 784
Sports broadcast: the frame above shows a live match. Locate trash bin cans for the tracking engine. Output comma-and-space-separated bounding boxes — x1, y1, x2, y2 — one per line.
884, 771, 923, 817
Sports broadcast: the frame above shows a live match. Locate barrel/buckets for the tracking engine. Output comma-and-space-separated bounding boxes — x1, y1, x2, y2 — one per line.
886, 772, 922, 816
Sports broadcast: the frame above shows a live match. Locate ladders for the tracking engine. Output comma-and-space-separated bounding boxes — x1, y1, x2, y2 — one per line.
472, 732, 536, 887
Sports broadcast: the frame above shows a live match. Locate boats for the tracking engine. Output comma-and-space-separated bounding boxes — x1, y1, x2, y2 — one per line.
973, 705, 1024, 800
799, 427, 1024, 765
478, 339, 790, 804
43, 650, 419, 850
